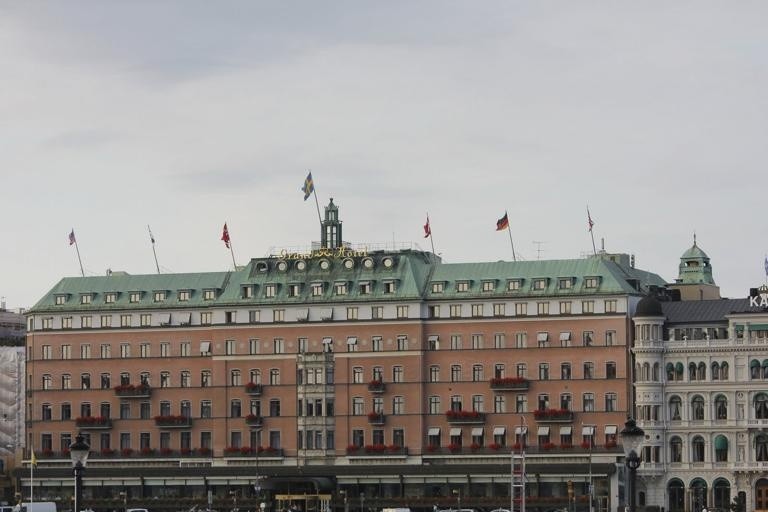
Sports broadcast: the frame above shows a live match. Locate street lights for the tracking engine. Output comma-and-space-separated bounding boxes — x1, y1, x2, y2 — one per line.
618, 419, 644, 512
69, 432, 90, 512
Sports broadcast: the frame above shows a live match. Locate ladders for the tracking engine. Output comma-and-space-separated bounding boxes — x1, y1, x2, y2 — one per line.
511, 451, 526, 512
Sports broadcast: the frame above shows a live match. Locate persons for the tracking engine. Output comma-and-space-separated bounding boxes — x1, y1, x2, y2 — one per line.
432, 503, 440, 512
326, 505, 332, 512
69, 493, 75, 512
231, 499, 302, 512
188, 504, 217, 512
367, 504, 377, 512
695, 496, 711, 512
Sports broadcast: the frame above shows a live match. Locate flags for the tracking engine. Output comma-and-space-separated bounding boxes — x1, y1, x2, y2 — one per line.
302, 173, 314, 200
586, 209, 594, 231
221, 224, 231, 249
69, 231, 75, 245
148, 226, 155, 243
496, 212, 509, 231
31, 449, 37, 466
423, 219, 431, 238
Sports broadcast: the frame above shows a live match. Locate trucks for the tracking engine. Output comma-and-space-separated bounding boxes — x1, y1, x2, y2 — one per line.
13, 502, 57, 512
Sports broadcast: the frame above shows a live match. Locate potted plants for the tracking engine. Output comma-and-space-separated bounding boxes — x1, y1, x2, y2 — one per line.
62, 447, 69, 455
153, 414, 192, 426
470, 443, 480, 454
368, 380, 385, 391
223, 446, 277, 457
123, 447, 133, 457
427, 444, 437, 454
245, 382, 256, 393
561, 444, 573, 448
448, 444, 458, 454
181, 448, 190, 456
113, 384, 151, 396
489, 376, 529, 388
159, 447, 173, 456
489, 444, 500, 449
101, 447, 113, 455
246, 415, 257, 424
142, 448, 150, 456
512, 443, 525, 454
368, 412, 385, 423
543, 442, 555, 449
581, 441, 594, 449
43, 447, 52, 456
445, 409, 485, 421
76, 416, 108, 427
346, 444, 402, 456
534, 407, 573, 420
